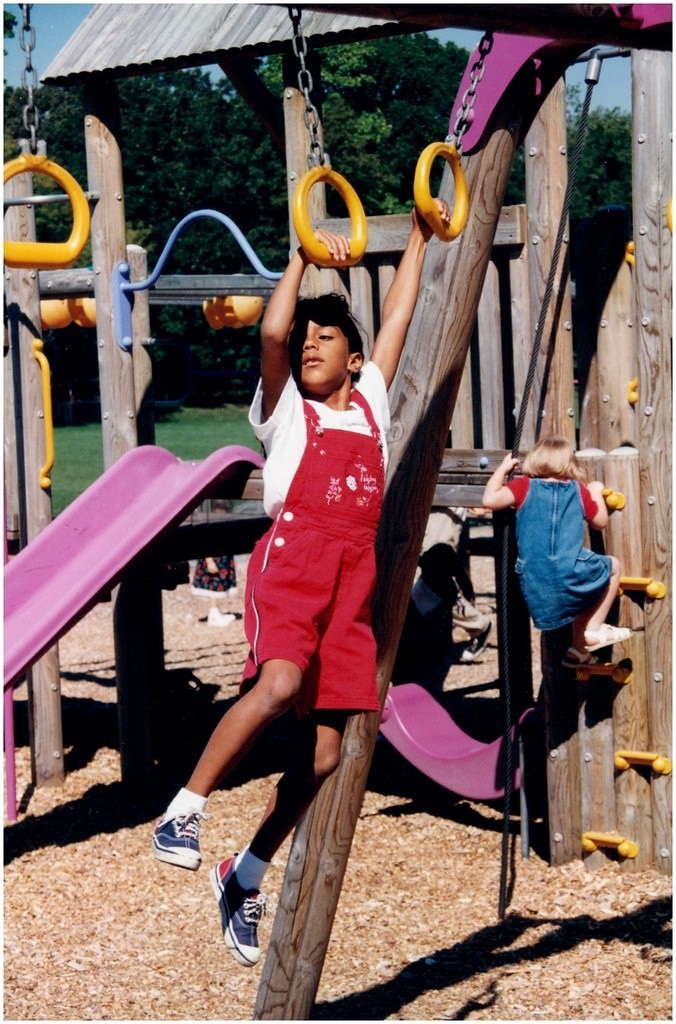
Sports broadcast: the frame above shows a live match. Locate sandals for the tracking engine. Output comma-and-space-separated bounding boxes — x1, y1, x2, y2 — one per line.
576, 623, 633, 652
561, 647, 600, 668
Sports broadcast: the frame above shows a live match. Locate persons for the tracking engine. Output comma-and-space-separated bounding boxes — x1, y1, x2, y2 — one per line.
189, 500, 236, 627
482, 438, 631, 667
151, 198, 450, 967
391, 507, 494, 692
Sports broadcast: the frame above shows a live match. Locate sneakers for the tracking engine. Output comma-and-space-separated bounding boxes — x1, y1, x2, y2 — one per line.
209, 853, 268, 967
151, 808, 202, 871
462, 621, 493, 660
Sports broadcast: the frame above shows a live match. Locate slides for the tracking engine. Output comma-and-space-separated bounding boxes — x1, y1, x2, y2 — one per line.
4, 444, 265, 694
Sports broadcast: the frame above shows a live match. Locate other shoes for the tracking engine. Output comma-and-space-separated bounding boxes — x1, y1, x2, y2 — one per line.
208, 608, 236, 627
